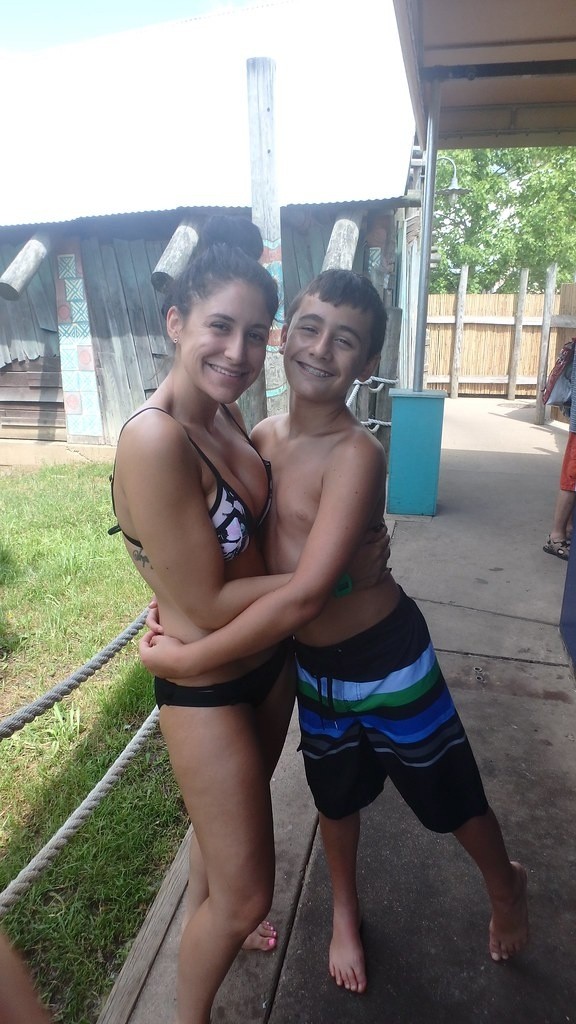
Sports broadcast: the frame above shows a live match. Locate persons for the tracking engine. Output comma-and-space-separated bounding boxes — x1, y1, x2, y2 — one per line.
106, 213, 393, 1024
0, 924, 58, 1024
543, 336, 576, 560
135, 264, 528, 990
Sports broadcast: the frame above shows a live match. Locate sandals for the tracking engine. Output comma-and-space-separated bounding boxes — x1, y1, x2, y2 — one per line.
541, 533, 571, 562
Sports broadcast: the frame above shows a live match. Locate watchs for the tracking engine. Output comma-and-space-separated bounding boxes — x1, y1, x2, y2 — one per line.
333, 573, 354, 598
